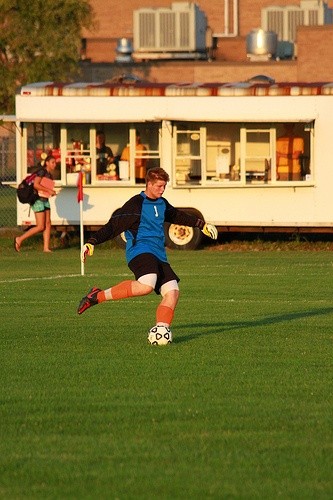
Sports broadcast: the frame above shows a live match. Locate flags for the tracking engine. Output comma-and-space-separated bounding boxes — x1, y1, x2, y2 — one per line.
77, 171, 84, 203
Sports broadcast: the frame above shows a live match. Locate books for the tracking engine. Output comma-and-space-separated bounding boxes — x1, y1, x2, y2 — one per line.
38, 177, 55, 197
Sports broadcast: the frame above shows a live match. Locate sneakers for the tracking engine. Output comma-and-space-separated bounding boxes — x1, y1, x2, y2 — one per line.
78, 287, 102, 315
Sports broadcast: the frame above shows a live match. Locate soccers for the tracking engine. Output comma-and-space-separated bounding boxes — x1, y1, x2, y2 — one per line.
148, 324, 172, 347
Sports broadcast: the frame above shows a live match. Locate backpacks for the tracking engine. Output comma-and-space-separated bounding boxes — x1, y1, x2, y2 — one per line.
17, 168, 46, 206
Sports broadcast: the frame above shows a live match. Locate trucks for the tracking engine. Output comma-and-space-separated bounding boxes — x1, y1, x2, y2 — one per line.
0, 82, 333, 250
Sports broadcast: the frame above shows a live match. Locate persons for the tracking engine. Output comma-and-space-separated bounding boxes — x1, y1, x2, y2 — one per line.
13, 156, 58, 253
120, 132, 146, 184
77, 167, 218, 329
96, 130, 113, 175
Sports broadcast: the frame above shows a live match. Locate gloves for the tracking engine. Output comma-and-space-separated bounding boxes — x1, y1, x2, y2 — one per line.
80, 243, 94, 263
201, 223, 218, 240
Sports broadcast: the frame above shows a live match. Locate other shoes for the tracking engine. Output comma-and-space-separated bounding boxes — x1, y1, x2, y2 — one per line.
14, 236, 20, 253
